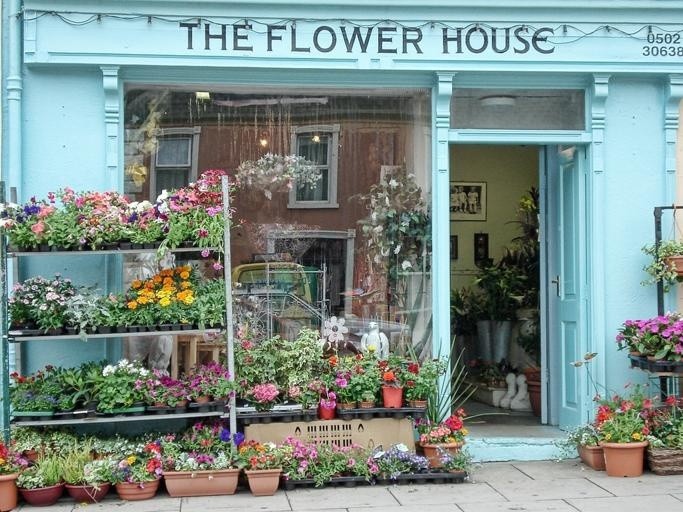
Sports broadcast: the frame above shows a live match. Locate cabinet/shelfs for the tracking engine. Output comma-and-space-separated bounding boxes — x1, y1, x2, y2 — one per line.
1, 171, 239, 450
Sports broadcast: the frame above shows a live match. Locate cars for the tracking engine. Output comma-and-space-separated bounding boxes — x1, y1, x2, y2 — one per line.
323, 316, 416, 359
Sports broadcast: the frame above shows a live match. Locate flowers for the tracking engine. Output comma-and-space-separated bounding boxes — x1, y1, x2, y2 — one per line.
236, 325, 382, 416
0, 442, 19, 474
376, 443, 467, 473
376, 355, 404, 385
414, 407, 468, 440
61, 449, 110, 482
286, 437, 375, 475
0, 169, 226, 254
160, 423, 244, 471
551, 309, 682, 479
109, 438, 162, 477
23, 455, 60, 488
404, 358, 441, 401
3, 361, 228, 418
238, 440, 291, 467
3, 265, 226, 336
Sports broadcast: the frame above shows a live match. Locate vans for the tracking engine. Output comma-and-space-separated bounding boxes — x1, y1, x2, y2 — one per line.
226, 253, 313, 332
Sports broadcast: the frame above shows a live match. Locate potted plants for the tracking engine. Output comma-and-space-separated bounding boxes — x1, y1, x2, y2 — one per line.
450, 187, 541, 416
639, 235, 682, 292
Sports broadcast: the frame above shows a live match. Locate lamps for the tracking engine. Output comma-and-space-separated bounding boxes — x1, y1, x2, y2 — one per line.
478, 95, 515, 114
258, 100, 322, 149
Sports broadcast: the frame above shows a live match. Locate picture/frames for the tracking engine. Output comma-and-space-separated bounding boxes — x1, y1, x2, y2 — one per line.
449, 180, 487, 222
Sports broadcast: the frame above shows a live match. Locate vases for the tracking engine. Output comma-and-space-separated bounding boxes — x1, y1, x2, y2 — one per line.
164, 468, 239, 496
0, 472, 18, 510
115, 479, 159, 499
377, 468, 467, 484
381, 386, 402, 408
19, 483, 63, 505
66, 482, 108, 503
419, 441, 467, 467
284, 478, 367, 488
406, 397, 427, 410
243, 466, 282, 496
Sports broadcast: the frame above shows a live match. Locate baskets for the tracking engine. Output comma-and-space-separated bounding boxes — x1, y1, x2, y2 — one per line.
647, 447, 683, 475
23, 446, 45, 463
337, 403, 356, 410
357, 401, 375, 409
